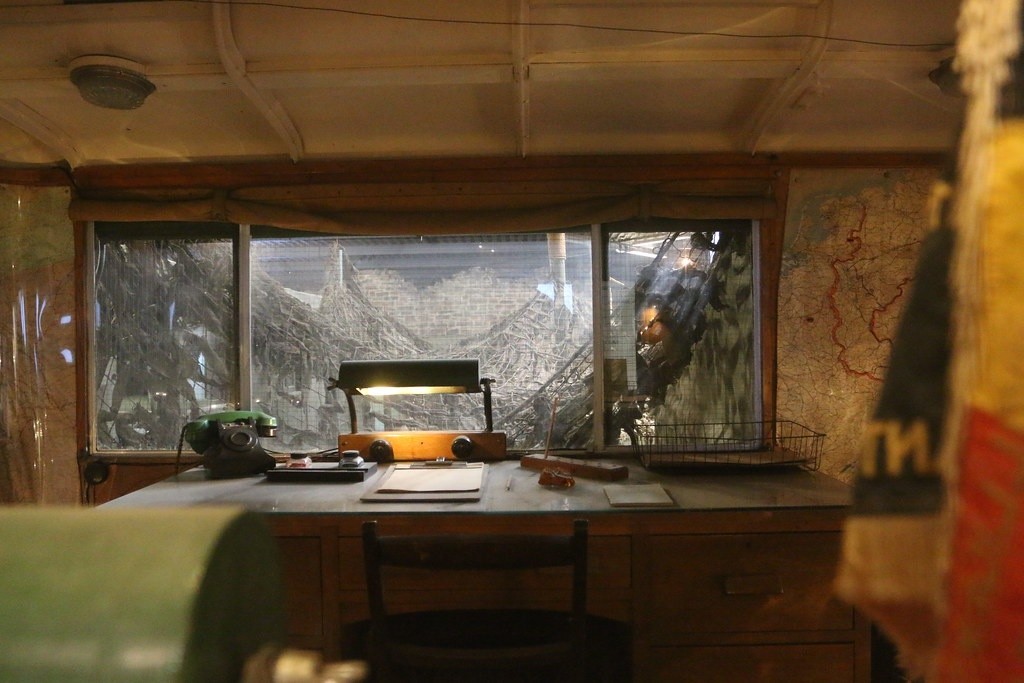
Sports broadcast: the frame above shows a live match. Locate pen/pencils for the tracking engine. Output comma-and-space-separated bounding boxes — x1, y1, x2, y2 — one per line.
505, 473, 513, 491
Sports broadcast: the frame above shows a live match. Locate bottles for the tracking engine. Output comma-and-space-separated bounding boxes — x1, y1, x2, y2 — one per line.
338, 449, 365, 469
286, 452, 312, 468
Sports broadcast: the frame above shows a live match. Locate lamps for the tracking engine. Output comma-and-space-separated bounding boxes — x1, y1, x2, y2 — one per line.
330, 359, 507, 462
69, 54, 155, 112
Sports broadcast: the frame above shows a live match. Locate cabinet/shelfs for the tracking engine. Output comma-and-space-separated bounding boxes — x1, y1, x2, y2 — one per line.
90, 456, 870, 683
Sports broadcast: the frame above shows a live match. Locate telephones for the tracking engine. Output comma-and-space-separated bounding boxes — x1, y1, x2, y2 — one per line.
183, 410, 278, 481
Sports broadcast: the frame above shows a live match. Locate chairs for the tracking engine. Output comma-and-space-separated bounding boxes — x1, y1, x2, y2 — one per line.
362, 518, 588, 683
